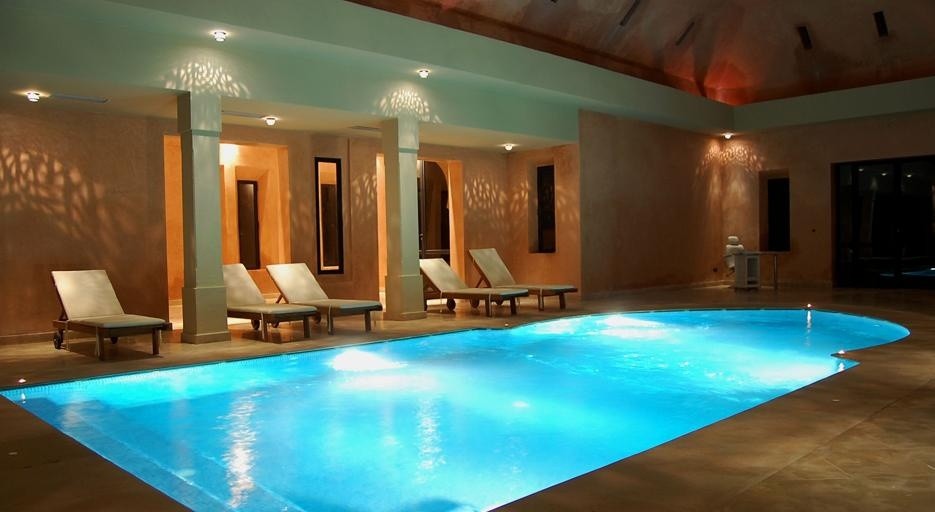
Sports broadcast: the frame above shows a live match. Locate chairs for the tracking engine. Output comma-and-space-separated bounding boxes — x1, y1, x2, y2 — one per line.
220, 261, 383, 340
419, 247, 579, 318
726, 236, 745, 289
48, 268, 173, 360
